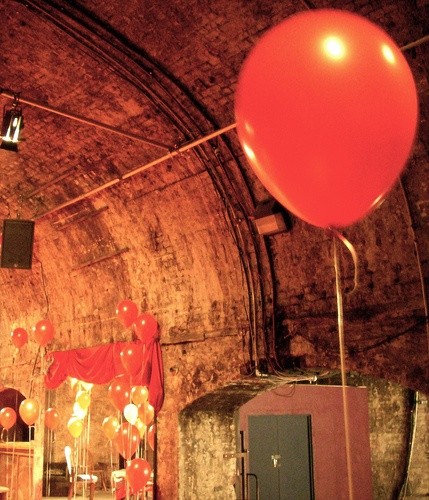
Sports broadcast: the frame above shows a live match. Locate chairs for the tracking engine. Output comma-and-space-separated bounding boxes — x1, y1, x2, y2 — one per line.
64, 445, 98, 500
110, 469, 154, 500
89, 455, 108, 492
68, 445, 95, 500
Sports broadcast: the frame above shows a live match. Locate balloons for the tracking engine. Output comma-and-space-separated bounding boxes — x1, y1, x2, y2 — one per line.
0, 301, 160, 497
233, 7, 417, 235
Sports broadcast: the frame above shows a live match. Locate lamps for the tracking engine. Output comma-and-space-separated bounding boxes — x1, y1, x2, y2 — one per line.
0, 89, 28, 153
251, 197, 296, 237
